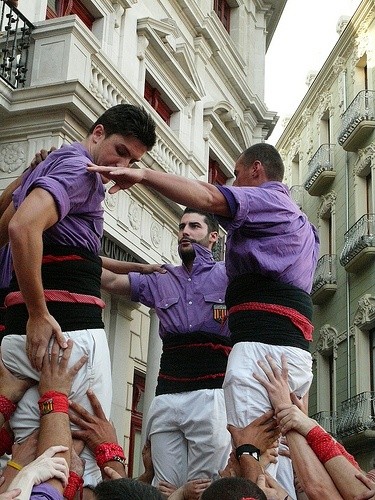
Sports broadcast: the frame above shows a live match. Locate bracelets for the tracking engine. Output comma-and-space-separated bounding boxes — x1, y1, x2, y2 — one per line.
284, 494, 290, 500
236, 444, 260, 461
7, 461, 23, 471
307, 426, 342, 464
38, 391, 69, 417
0, 394, 17, 420
115, 456, 125, 468
96, 442, 126, 469
63, 471, 83, 500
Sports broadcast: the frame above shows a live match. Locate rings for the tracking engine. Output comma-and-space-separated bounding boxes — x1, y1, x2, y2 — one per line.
142, 452, 145, 455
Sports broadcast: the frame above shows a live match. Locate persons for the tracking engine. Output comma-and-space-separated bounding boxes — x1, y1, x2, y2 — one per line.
0, 105, 156, 500
252, 353, 375, 500
100, 206, 231, 489
0, 146, 58, 344
85, 143, 319, 500
0, 335, 297, 500
